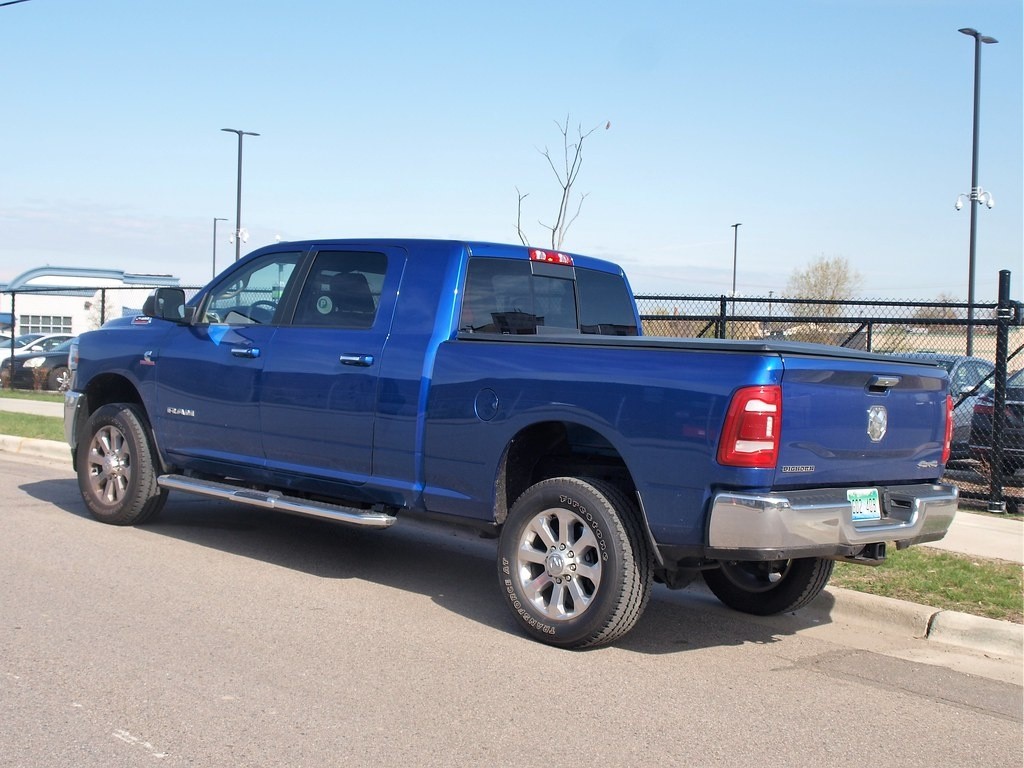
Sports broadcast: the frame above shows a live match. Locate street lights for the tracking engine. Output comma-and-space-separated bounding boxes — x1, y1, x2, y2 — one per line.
959, 28, 998, 355
213, 219, 229, 279
732, 222, 742, 339
222, 128, 261, 307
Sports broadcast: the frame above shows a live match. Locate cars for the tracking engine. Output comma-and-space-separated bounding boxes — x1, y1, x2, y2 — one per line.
0, 333, 77, 391
968, 367, 1024, 477
882, 351, 996, 459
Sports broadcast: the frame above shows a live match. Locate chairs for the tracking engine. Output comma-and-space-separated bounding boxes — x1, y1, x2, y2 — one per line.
318, 269, 376, 319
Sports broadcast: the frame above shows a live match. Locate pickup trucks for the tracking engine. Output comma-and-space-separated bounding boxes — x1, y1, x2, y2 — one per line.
62, 238, 960, 652
